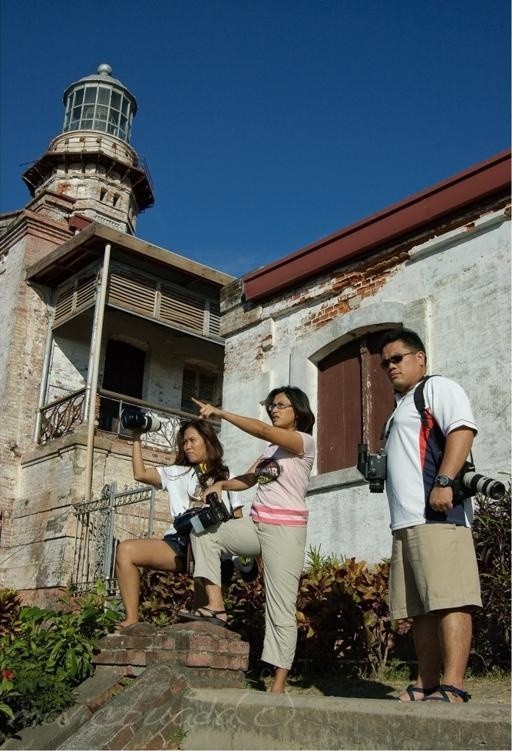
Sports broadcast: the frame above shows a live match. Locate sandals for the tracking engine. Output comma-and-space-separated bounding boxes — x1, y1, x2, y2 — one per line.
396, 684, 471, 704
175, 607, 228, 627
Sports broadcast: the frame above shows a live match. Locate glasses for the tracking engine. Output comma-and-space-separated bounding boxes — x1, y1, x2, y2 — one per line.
268, 403, 293, 412
380, 351, 416, 369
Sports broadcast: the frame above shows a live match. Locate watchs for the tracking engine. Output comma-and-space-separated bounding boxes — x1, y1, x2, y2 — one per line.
434, 474, 453, 489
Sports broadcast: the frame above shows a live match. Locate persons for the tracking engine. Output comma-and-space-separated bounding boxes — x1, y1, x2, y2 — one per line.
377, 329, 485, 704
176, 384, 316, 694
114, 418, 244, 635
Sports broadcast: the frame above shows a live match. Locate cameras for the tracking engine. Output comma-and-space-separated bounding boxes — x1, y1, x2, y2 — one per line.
452, 460, 506, 501
190, 493, 231, 534
120, 408, 162, 433
356, 442, 388, 494
